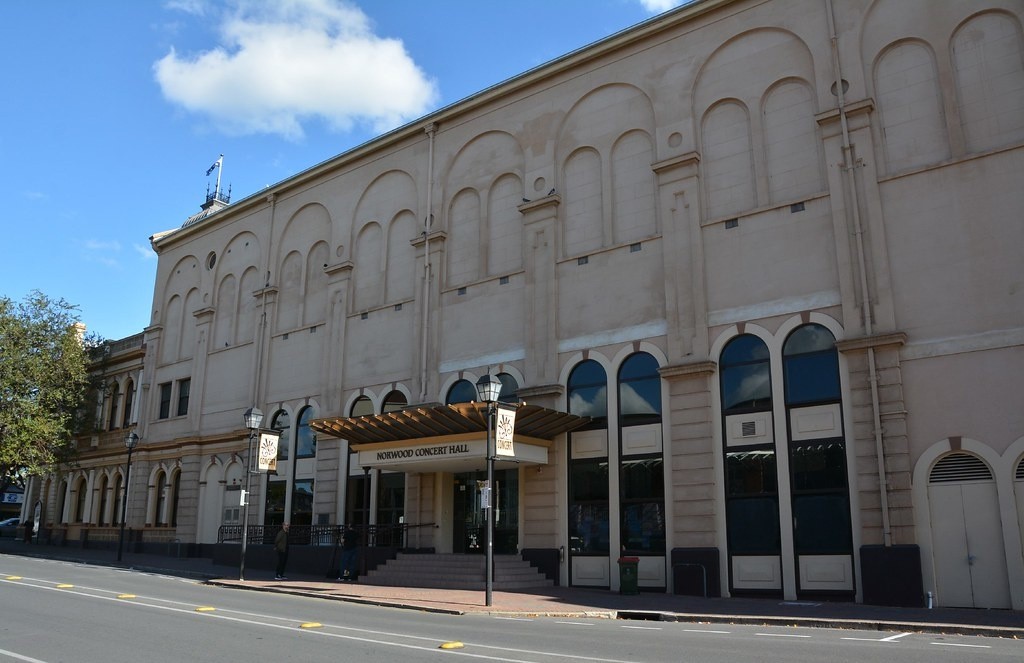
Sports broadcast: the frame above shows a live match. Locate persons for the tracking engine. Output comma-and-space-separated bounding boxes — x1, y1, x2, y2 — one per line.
21, 516, 34, 545
272, 522, 290, 581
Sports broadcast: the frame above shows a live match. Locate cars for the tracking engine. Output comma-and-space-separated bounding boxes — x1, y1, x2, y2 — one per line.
0, 518, 20, 539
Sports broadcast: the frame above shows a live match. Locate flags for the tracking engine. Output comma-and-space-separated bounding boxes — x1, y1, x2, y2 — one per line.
206, 159, 221, 177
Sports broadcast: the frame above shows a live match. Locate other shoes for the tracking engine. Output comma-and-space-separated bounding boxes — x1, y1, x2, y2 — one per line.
275, 576, 286, 581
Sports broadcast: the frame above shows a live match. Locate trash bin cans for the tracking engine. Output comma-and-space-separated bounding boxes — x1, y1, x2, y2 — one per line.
617, 557, 641, 596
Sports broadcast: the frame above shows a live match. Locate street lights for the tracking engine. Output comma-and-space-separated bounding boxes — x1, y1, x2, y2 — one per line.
238, 405, 262, 581
475, 364, 502, 607
115, 431, 139, 565
